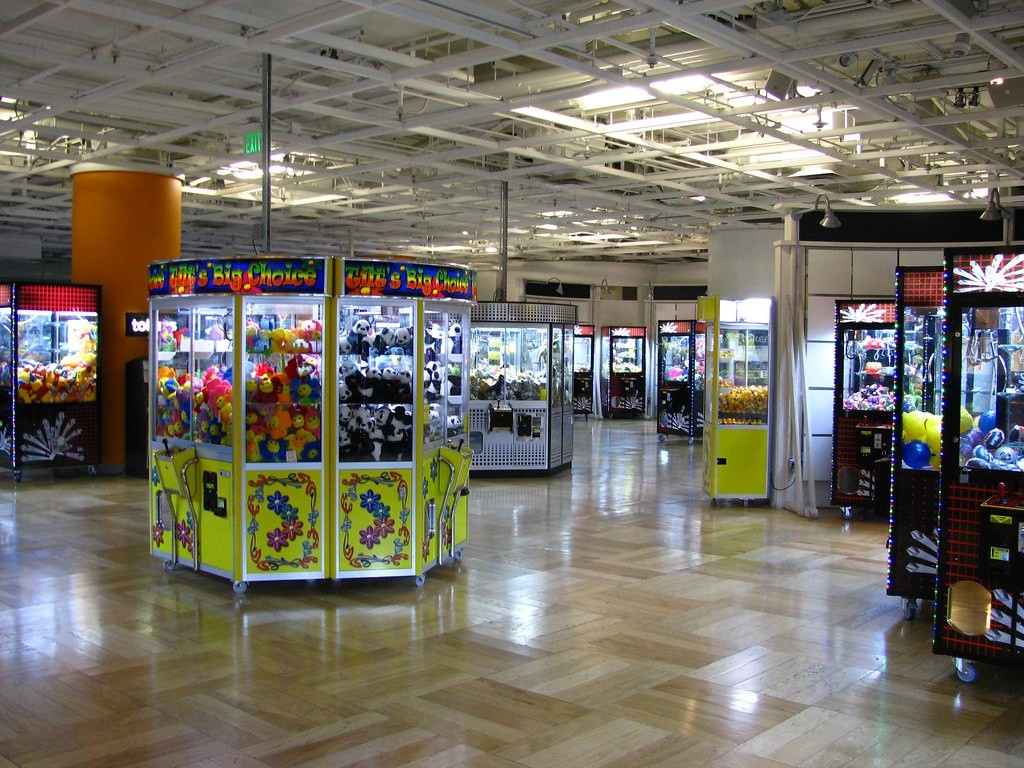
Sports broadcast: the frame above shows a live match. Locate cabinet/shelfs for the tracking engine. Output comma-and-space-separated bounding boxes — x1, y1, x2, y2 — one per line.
156, 295, 467, 463
843, 330, 923, 411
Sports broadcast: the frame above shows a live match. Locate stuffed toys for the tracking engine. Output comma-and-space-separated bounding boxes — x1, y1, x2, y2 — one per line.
718, 350, 768, 425
0, 319, 98, 404
156, 318, 462, 463
844, 335, 923, 412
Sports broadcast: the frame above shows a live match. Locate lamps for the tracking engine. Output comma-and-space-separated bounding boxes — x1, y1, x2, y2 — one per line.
590, 279, 611, 294
546, 277, 563, 295
979, 188, 1015, 221
790, 194, 842, 228
